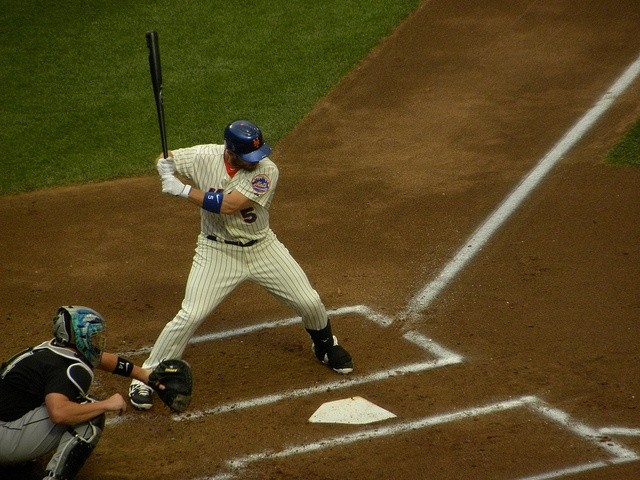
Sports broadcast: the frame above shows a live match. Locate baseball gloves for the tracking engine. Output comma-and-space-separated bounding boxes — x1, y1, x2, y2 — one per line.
148, 359, 192, 411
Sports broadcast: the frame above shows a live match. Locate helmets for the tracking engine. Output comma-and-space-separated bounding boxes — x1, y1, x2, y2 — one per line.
224, 120, 272, 162
52, 306, 106, 368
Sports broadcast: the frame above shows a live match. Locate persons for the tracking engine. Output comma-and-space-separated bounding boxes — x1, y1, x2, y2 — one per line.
128, 120, 354, 411
1, 304, 194, 480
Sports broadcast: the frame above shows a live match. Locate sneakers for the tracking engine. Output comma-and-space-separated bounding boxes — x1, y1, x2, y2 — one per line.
128, 384, 153, 411
311, 335, 353, 374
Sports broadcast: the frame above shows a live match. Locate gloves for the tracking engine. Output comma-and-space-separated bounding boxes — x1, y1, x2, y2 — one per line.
157, 157, 177, 176
160, 175, 192, 198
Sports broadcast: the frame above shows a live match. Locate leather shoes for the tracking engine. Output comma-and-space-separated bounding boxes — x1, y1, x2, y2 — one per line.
206, 234, 257, 246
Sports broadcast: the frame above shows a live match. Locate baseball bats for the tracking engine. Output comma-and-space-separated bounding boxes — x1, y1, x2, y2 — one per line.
146, 31, 168, 159
